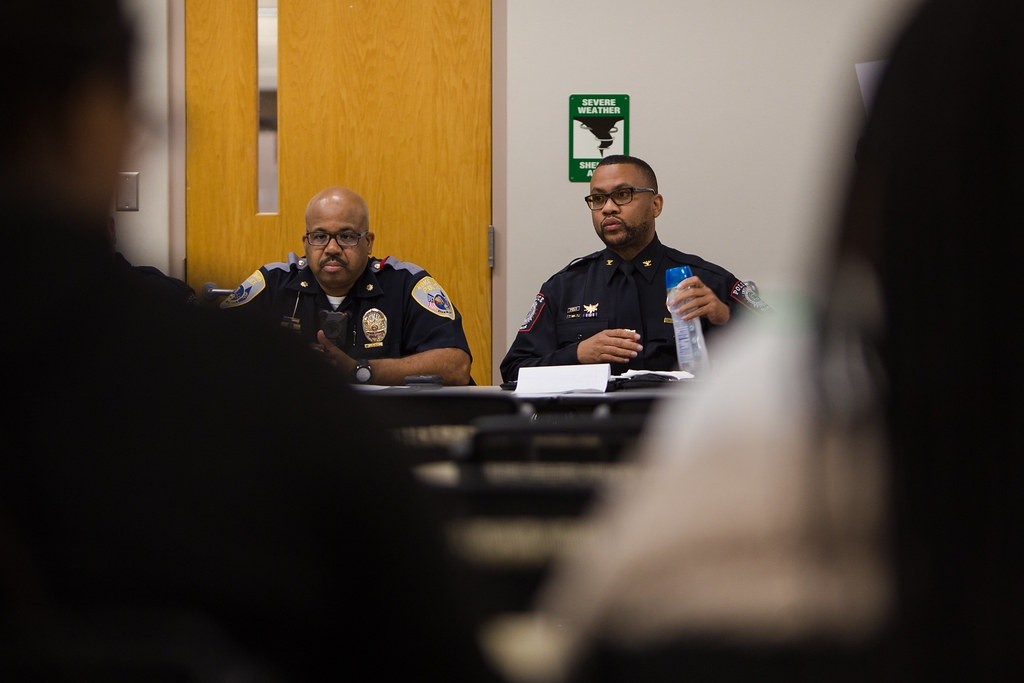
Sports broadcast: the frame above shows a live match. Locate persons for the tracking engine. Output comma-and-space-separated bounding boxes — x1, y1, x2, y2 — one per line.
498, 154, 782, 382
553, 0, 1024, 683
216, 187, 477, 387
0, 0, 498, 682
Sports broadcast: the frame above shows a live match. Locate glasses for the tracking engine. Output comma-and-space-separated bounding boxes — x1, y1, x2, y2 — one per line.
585, 187, 655, 210
306, 230, 368, 246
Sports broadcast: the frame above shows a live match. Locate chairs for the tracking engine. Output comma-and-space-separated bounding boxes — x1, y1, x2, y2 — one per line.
362, 388, 673, 607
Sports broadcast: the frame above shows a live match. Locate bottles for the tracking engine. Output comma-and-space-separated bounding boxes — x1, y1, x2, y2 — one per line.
666, 265, 708, 371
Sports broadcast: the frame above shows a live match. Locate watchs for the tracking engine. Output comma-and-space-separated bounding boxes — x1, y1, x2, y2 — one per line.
353, 357, 373, 384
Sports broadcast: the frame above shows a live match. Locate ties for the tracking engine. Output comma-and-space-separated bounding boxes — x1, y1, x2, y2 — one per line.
615, 265, 644, 373
